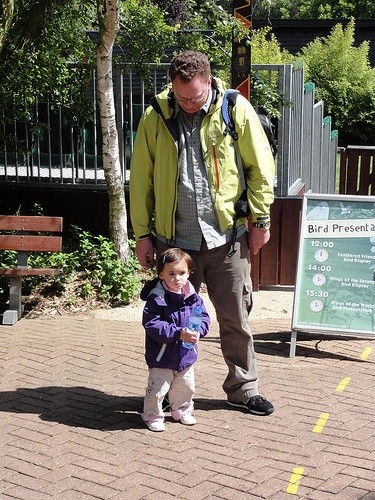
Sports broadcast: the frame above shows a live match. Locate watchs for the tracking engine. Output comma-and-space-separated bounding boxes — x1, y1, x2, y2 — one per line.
252, 221, 271, 232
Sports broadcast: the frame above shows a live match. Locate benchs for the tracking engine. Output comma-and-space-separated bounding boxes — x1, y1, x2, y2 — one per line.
0, 215, 63, 325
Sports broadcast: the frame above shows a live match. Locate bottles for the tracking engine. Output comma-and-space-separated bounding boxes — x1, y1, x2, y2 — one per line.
182, 307, 203, 349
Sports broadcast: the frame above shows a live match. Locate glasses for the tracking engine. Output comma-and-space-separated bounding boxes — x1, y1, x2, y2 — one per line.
172, 84, 208, 102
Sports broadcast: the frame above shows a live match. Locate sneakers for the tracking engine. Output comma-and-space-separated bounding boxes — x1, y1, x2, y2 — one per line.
146, 418, 165, 432
161, 397, 171, 411
180, 412, 197, 425
227, 395, 274, 415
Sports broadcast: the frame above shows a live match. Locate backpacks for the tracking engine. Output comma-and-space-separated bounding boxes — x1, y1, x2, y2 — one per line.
223, 88, 279, 218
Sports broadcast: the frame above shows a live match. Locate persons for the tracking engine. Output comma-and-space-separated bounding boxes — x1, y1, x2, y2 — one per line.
129, 51, 277, 415
140, 247, 211, 431
